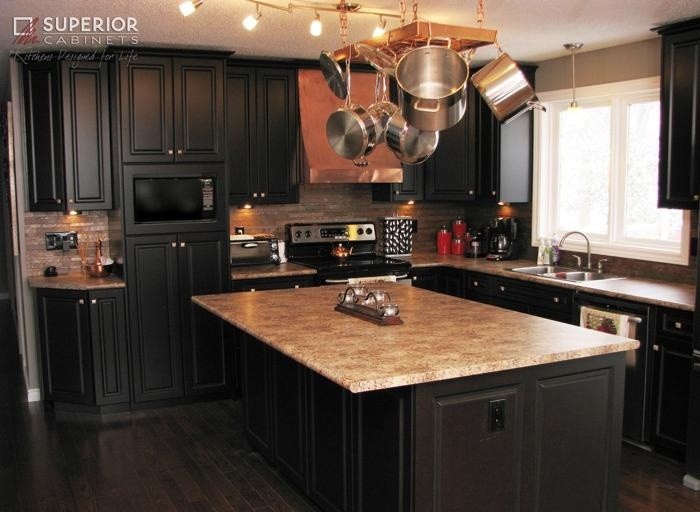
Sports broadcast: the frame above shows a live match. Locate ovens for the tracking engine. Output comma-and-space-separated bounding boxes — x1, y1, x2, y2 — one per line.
228, 234, 279, 266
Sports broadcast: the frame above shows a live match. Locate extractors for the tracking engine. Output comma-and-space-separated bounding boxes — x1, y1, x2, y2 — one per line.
298, 66, 407, 185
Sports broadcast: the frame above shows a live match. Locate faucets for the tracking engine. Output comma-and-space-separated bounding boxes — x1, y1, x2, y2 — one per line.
559, 231, 593, 270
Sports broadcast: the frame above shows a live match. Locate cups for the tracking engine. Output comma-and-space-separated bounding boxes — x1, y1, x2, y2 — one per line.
341, 279, 397, 318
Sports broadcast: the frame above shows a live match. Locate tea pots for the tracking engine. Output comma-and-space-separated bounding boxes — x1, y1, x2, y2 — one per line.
330, 236, 354, 260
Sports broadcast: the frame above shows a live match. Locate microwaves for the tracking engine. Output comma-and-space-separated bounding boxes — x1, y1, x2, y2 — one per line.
134, 177, 216, 223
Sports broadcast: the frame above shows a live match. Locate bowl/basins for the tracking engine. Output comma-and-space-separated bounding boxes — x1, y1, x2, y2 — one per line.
85, 257, 114, 278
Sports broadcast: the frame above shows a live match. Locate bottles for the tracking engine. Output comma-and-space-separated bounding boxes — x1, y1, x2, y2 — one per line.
437, 213, 472, 256
91, 240, 105, 278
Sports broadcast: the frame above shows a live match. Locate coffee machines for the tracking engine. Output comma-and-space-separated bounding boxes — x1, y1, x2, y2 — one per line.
487, 215, 518, 261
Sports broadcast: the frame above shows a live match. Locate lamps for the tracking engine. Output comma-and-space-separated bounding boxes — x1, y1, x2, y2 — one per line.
179, 0, 402, 39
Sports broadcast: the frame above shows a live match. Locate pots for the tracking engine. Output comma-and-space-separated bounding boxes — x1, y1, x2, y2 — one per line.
324, 42, 548, 167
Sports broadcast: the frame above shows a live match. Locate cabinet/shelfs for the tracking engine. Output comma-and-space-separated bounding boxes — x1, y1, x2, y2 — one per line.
126, 231, 230, 404
225, 66, 299, 204
121, 55, 224, 163
424, 68, 534, 202
22, 61, 113, 212
658, 30, 700, 210
372, 162, 423, 201
36, 287, 130, 406
231, 265, 700, 479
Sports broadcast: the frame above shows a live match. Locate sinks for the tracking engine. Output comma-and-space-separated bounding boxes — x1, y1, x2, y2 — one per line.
503, 265, 585, 275
539, 270, 633, 284
